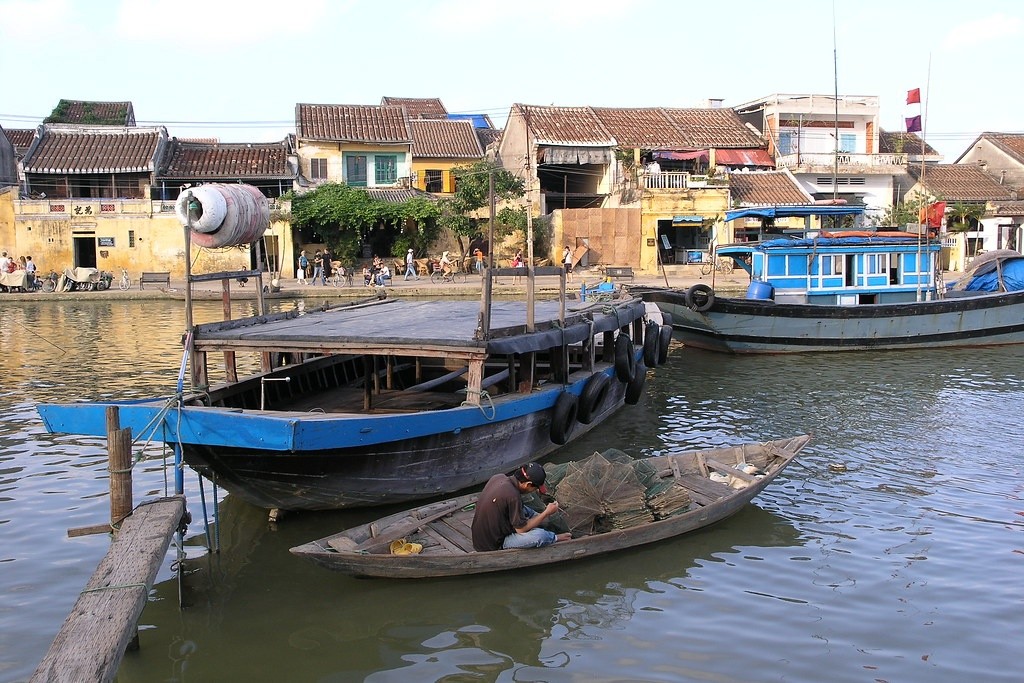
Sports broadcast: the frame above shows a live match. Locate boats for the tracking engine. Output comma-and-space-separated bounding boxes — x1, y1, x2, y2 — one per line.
287, 435, 813, 579
615, 50, 1024, 354
36, 172, 675, 512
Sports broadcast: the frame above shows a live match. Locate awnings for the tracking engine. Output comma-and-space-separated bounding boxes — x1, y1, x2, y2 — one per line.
724, 209, 776, 222
655, 149, 775, 166
672, 216, 703, 228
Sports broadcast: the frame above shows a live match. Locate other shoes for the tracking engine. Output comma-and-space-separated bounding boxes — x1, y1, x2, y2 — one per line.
375, 285, 379, 287
380, 284, 385, 287
296, 281, 302, 284
404, 278, 409, 281
305, 281, 309, 284
415, 276, 420, 281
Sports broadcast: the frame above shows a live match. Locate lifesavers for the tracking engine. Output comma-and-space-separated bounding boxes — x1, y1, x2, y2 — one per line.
645, 312, 673, 368
615, 331, 645, 405
685, 284, 715, 313
550, 373, 611, 446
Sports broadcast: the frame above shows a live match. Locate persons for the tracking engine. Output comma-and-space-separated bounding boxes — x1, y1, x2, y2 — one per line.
472, 461, 572, 549
404, 248, 420, 281
238, 266, 249, 287
563, 246, 573, 284
473, 247, 482, 277
438, 251, 453, 277
0, 252, 34, 293
298, 249, 308, 285
512, 248, 524, 285
312, 248, 331, 286
368, 254, 389, 285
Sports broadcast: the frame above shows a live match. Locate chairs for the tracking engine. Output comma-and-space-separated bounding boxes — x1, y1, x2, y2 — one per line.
393, 258, 430, 276
330, 260, 340, 275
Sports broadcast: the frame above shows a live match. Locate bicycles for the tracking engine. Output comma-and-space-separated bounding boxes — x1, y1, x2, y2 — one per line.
118, 266, 131, 291
430, 264, 467, 285
0, 266, 114, 293
701, 254, 733, 275
331, 264, 355, 288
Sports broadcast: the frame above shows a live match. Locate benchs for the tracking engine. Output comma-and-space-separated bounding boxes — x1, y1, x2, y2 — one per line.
140, 272, 170, 290
604, 267, 635, 283
706, 457, 755, 482
363, 268, 394, 285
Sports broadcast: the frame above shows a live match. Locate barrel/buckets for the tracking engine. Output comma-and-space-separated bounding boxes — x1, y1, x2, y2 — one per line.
745, 279, 773, 298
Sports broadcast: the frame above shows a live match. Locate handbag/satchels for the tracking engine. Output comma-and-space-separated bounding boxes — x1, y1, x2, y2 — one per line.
511, 259, 519, 267
297, 269, 304, 279
561, 258, 566, 263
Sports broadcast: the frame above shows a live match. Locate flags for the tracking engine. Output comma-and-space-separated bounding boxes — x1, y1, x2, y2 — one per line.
907, 115, 922, 132
906, 89, 920, 105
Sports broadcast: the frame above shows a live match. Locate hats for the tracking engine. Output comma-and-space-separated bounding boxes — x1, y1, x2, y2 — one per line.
522, 462, 548, 494
408, 248, 413, 252
443, 250, 449, 257
